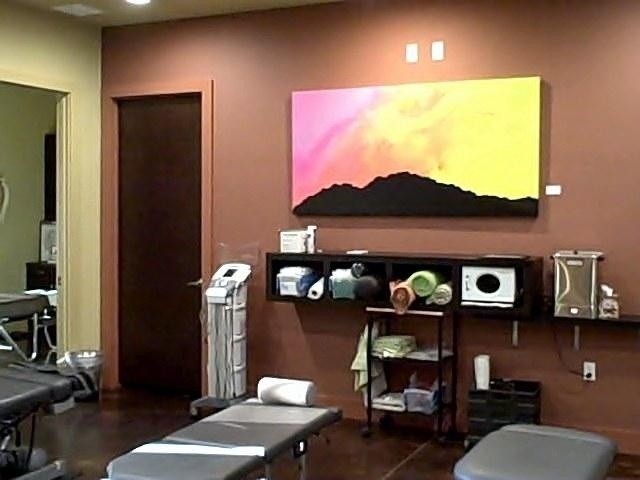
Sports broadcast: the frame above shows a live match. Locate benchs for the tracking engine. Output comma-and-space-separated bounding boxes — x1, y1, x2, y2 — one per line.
0, 364, 73, 480
105, 397, 343, 480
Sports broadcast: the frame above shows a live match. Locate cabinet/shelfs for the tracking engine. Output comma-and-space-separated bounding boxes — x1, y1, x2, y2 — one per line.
27, 262, 56, 363
265, 251, 543, 445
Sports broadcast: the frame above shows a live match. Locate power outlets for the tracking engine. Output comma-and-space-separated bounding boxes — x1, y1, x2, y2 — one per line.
583, 361, 596, 381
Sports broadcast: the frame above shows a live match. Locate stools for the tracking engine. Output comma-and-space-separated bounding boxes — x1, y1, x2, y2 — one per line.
455, 423, 616, 479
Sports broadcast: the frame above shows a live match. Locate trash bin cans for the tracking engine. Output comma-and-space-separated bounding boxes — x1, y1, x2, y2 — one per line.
67, 350, 104, 403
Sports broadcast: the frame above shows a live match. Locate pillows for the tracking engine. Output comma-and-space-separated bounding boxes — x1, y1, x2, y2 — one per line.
257, 376, 314, 406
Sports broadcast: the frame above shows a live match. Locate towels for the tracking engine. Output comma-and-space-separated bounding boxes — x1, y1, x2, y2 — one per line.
349, 269, 453, 407
